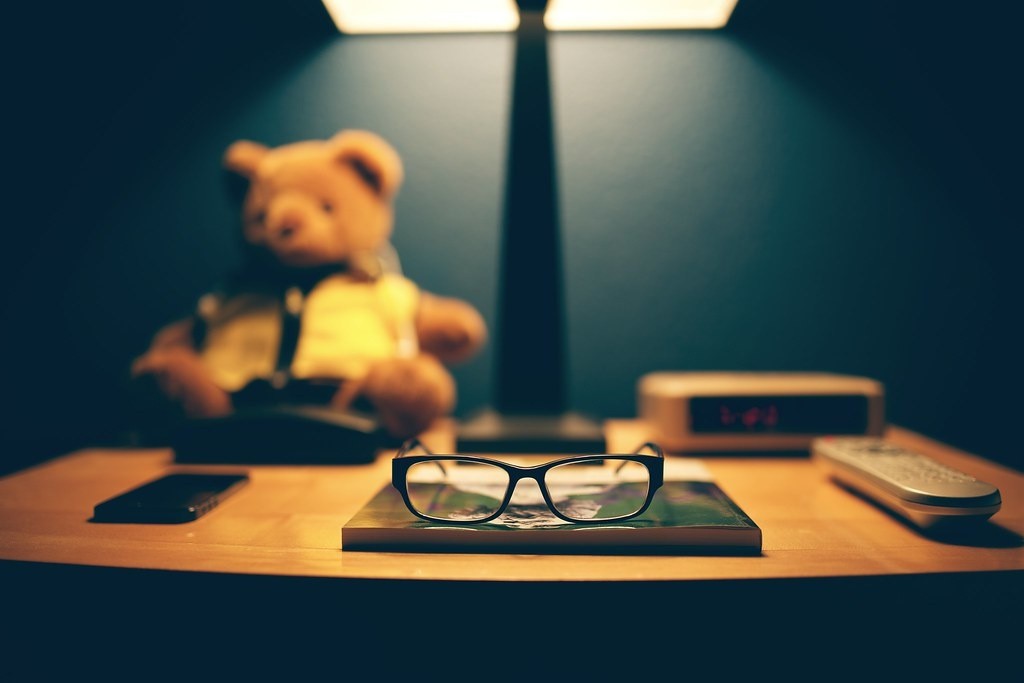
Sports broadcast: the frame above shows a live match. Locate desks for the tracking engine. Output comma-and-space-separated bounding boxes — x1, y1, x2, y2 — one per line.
3, 411, 1023, 591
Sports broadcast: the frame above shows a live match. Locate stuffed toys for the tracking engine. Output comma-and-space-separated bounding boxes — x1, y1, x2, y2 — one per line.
132, 130, 487, 443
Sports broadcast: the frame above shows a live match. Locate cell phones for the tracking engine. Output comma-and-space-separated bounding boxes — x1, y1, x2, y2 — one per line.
93, 473, 249, 522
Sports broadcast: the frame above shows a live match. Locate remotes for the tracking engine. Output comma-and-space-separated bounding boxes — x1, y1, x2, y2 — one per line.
814, 436, 1002, 529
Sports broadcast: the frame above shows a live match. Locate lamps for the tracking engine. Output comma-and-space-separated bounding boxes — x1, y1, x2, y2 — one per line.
319, 0, 737, 465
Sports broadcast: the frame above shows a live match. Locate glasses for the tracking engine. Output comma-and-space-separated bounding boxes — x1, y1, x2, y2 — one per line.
391, 436, 665, 524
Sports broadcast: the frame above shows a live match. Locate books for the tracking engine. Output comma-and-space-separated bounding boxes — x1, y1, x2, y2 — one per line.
341, 454, 762, 557
